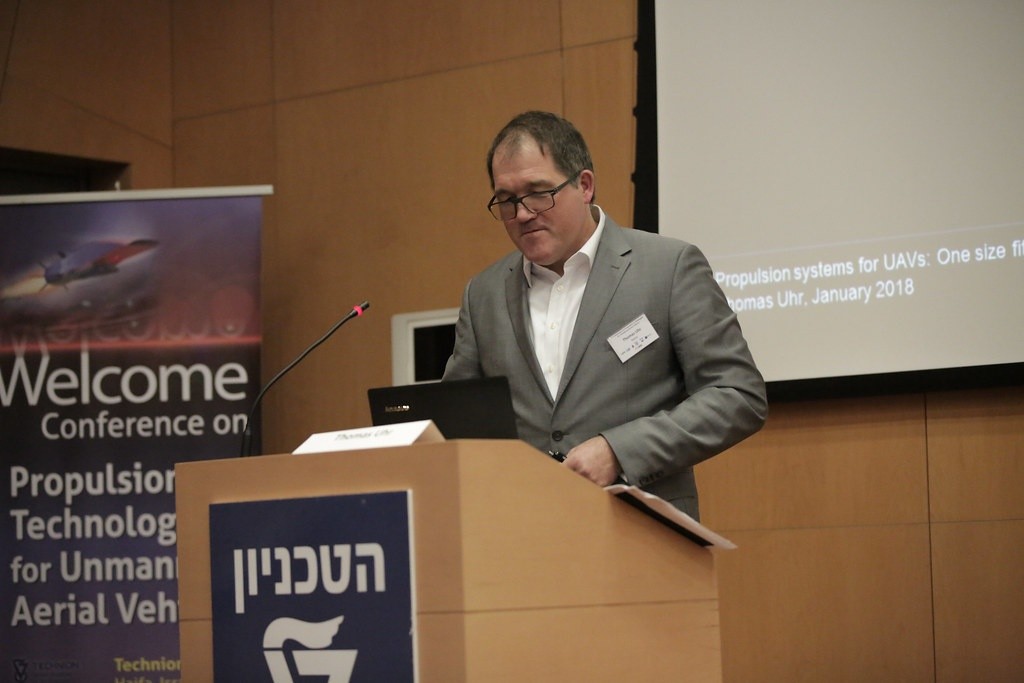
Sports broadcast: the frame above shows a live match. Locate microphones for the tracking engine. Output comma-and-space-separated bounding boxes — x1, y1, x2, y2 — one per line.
240, 302, 371, 457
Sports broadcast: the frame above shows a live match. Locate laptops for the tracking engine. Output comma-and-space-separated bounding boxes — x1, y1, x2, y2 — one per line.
369, 374, 518, 440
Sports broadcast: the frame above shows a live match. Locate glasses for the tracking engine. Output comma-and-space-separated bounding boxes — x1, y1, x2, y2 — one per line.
488, 170, 579, 222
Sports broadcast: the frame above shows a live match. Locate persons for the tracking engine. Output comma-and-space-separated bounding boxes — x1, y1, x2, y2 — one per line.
439, 111, 770, 525
